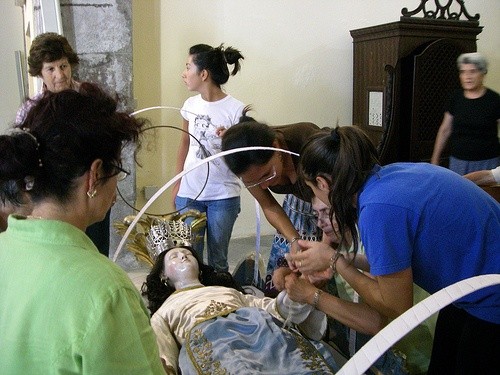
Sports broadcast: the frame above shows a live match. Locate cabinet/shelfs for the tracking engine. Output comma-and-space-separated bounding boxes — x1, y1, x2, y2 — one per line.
349, 0, 484, 167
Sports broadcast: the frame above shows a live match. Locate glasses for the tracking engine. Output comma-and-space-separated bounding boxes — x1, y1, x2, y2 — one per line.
79, 163, 130, 181
241, 163, 277, 188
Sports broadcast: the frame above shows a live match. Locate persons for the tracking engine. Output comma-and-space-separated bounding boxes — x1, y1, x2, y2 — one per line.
0, 89, 168, 374
461, 166, 500, 186
145, 248, 338, 375
220, 116, 500, 375
16, 32, 110, 256
169, 44, 246, 275
430, 52, 500, 175
